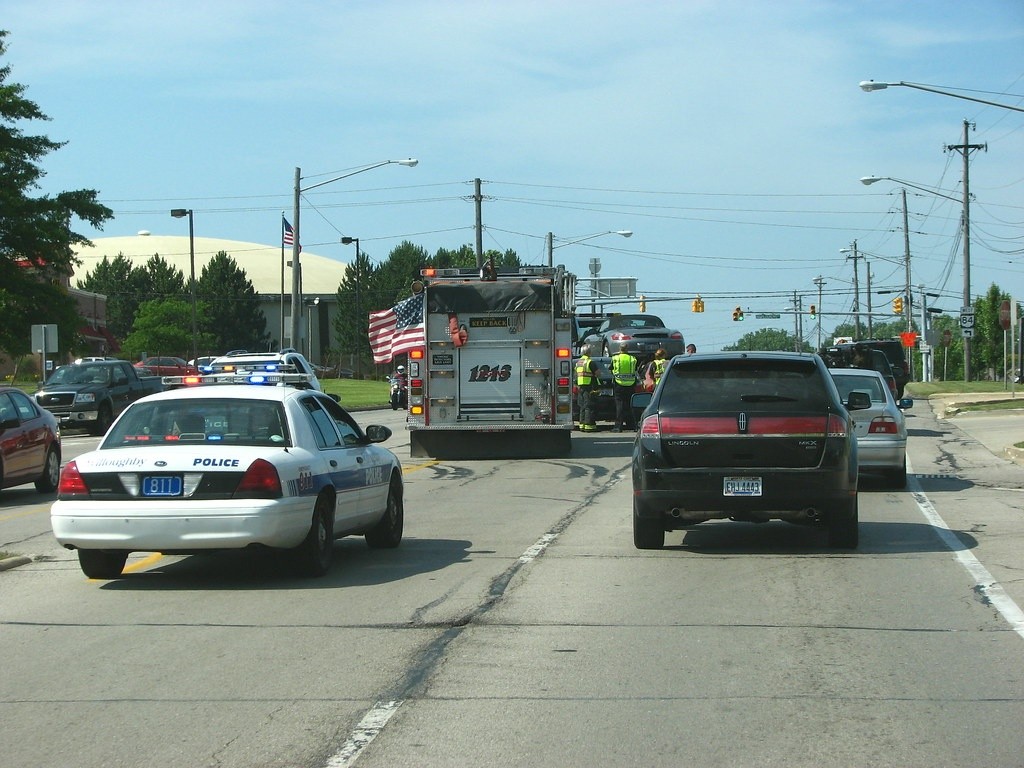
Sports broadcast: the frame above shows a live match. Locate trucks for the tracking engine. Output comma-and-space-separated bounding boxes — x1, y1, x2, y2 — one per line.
571, 356, 669, 422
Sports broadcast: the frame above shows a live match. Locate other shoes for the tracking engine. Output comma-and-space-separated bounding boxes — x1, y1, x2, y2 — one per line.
580, 428, 602, 432
610, 427, 622, 433
634, 428, 638, 432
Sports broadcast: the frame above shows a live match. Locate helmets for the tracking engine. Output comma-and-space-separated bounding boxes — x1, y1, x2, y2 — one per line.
619, 342, 629, 352
581, 344, 592, 355
397, 365, 405, 374
656, 349, 667, 358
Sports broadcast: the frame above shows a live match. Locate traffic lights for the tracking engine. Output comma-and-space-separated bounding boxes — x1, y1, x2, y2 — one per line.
810, 305, 816, 320
735, 306, 741, 311
733, 312, 738, 321
892, 296, 902, 313
738, 311, 743, 321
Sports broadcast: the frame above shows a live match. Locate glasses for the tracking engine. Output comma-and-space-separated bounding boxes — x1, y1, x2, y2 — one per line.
687, 349, 690, 352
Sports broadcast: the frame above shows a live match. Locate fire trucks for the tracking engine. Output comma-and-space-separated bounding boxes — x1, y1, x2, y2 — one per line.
404, 264, 579, 461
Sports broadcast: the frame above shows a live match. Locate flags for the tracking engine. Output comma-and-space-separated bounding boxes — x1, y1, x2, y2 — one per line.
283, 218, 301, 253
368, 292, 426, 364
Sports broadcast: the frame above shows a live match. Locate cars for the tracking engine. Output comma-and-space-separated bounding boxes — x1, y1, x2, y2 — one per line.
0, 386, 62, 496
827, 366, 914, 489
580, 313, 686, 365
56, 345, 322, 407
50, 381, 404, 582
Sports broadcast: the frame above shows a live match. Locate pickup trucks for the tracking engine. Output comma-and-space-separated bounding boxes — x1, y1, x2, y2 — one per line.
33, 354, 178, 436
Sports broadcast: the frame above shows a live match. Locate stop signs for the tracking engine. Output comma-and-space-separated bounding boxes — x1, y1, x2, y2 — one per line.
998, 300, 1011, 330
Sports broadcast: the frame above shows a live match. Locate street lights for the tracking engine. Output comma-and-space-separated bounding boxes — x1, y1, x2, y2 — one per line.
839, 248, 914, 382
814, 282, 828, 352
859, 176, 973, 384
784, 307, 803, 351
290, 158, 419, 351
170, 208, 198, 374
341, 236, 360, 381
547, 230, 634, 269
812, 277, 860, 341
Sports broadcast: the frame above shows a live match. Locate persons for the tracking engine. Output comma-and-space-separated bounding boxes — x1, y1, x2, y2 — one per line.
686, 344, 696, 354
600, 343, 646, 433
388, 366, 408, 410
849, 343, 865, 369
637, 348, 671, 401
574, 344, 600, 431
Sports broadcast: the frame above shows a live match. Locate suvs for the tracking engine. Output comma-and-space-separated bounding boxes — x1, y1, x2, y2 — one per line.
815, 338, 911, 401
629, 349, 872, 551
